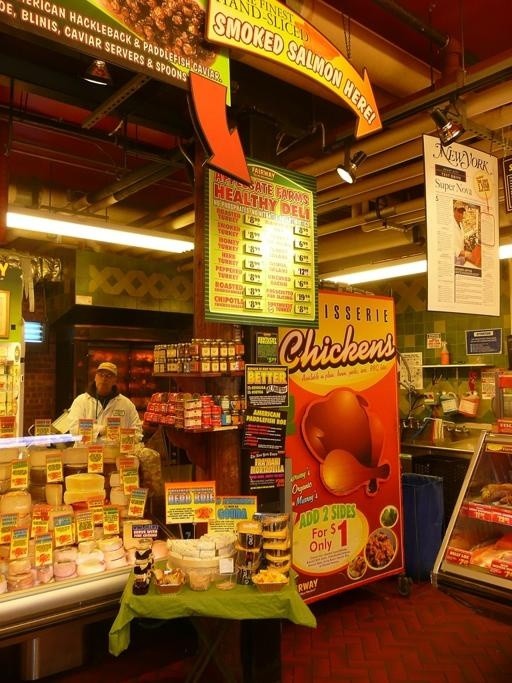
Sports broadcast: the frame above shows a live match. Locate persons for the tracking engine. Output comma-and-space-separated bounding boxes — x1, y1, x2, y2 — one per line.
66, 361, 143, 438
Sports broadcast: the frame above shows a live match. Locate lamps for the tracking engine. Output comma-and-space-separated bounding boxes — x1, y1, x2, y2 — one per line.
428, 104, 466, 147
24, 320, 48, 345
80, 58, 111, 87
5, 162, 197, 256
335, 150, 369, 184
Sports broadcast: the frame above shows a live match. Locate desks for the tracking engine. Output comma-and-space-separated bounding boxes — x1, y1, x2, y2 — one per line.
107, 557, 317, 681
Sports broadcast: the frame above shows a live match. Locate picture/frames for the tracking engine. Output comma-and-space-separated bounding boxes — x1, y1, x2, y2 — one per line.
1, 287, 11, 339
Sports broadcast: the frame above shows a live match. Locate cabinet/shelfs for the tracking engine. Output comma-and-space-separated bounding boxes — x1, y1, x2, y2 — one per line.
142, 370, 244, 435
430, 425, 511, 620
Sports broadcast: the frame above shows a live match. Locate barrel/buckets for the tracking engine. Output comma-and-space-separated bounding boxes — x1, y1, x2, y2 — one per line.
458, 389, 480, 418
439, 392, 458, 414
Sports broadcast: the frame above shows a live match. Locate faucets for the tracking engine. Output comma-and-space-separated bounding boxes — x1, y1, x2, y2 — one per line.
422, 416, 445, 423
400, 379, 413, 391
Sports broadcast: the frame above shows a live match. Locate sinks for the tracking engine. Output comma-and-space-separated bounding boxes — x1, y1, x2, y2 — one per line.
400, 423, 485, 459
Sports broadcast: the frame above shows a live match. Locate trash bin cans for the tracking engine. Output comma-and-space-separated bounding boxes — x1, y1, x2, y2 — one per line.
393, 473, 449, 583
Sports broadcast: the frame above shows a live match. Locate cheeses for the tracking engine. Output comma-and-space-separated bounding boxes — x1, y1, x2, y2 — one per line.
0, 445, 237, 595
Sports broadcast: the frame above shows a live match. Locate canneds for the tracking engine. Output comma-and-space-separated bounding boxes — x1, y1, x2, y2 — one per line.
144, 391, 246, 429
153, 337, 246, 373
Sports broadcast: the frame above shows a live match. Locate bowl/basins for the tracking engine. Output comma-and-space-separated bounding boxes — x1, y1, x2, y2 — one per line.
347, 504, 398, 581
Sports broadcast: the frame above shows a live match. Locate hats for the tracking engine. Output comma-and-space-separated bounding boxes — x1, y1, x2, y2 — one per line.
97, 361, 118, 376
